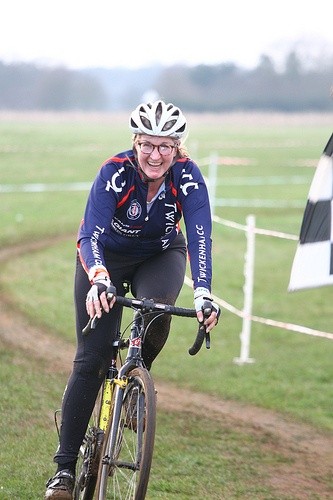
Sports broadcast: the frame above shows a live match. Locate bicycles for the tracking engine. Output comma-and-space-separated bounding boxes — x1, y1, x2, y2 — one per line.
55, 279, 214, 499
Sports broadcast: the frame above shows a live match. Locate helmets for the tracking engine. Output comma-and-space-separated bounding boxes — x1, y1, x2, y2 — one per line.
129, 100, 189, 146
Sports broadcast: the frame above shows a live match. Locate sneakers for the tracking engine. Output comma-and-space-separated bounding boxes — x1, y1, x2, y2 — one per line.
45, 468, 76, 500
124, 389, 146, 433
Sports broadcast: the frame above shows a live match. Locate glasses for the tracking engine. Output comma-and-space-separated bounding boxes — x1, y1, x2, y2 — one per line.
137, 141, 177, 155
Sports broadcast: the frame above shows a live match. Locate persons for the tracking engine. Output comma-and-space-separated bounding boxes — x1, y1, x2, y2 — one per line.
43, 99, 221, 500
